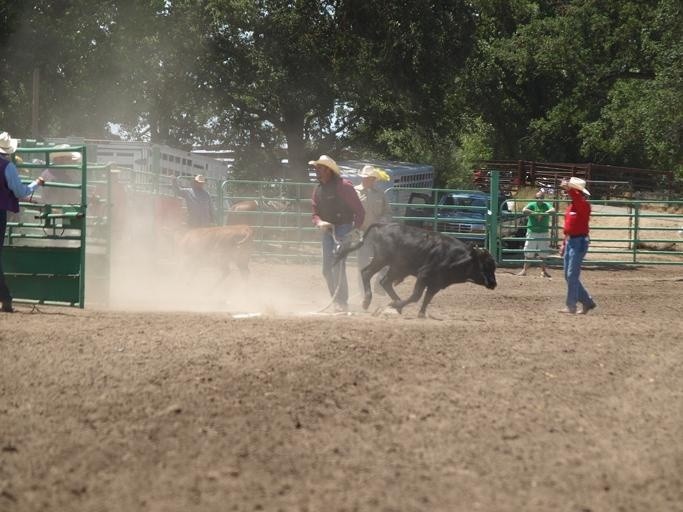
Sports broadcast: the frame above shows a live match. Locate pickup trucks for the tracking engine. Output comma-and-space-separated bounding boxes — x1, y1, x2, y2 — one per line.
398, 188, 529, 255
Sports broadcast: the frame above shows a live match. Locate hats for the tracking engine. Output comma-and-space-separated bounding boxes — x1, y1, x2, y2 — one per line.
49, 144, 80, 160
358, 165, 378, 177
194, 175, 204, 182
568, 177, 590, 196
308, 155, 339, 175
0, 132, 17, 154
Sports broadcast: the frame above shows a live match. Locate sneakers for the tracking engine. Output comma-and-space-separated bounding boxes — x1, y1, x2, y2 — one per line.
334, 303, 346, 312
540, 272, 550, 277
2, 297, 12, 311
518, 270, 526, 276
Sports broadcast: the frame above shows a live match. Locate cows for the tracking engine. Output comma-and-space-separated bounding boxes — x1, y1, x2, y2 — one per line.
329, 221, 499, 318
161, 222, 253, 282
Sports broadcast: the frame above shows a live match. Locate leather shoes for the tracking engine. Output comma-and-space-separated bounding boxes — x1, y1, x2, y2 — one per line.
558, 305, 575, 313
577, 302, 595, 313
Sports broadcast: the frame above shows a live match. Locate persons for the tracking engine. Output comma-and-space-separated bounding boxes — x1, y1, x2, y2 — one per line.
308, 154, 365, 310
166, 173, 215, 230
351, 165, 389, 297
515, 192, 557, 278
37, 143, 81, 205
556, 176, 598, 315
0, 131, 46, 316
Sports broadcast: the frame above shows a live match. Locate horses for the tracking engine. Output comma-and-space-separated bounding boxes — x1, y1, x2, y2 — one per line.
228, 199, 306, 230
